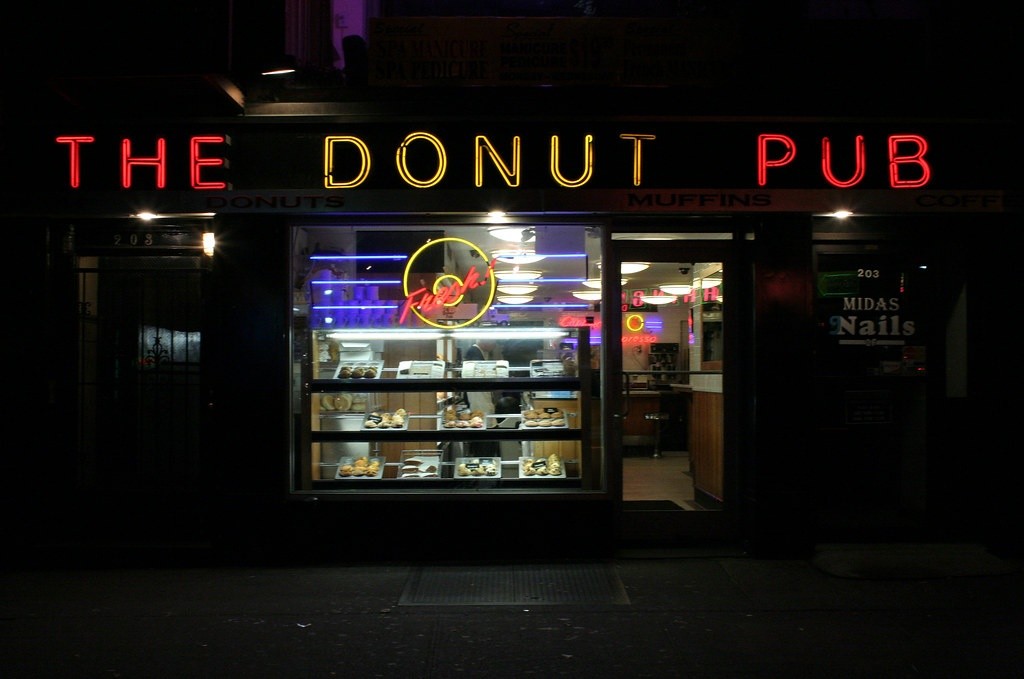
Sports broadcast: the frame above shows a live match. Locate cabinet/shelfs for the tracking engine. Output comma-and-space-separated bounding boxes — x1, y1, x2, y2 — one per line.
300, 324, 592, 487
647, 343, 678, 380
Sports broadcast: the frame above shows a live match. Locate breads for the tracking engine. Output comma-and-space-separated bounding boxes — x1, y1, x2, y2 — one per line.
319, 392, 367, 411
402, 458, 438, 474
524, 409, 565, 427
339, 456, 380, 477
560, 348, 577, 376
522, 453, 563, 475
337, 366, 377, 378
364, 407, 406, 428
442, 408, 484, 428
457, 463, 496, 476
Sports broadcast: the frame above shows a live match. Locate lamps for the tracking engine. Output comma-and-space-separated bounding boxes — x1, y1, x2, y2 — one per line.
641, 296, 678, 304
500, 296, 534, 306
485, 225, 537, 245
497, 284, 537, 294
573, 291, 603, 300
659, 282, 692, 295
324, 329, 571, 344
493, 270, 542, 281
692, 275, 722, 289
597, 261, 653, 274
489, 249, 546, 264
585, 278, 627, 289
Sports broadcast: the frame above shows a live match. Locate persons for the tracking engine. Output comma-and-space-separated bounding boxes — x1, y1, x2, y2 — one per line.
463, 339, 502, 458
